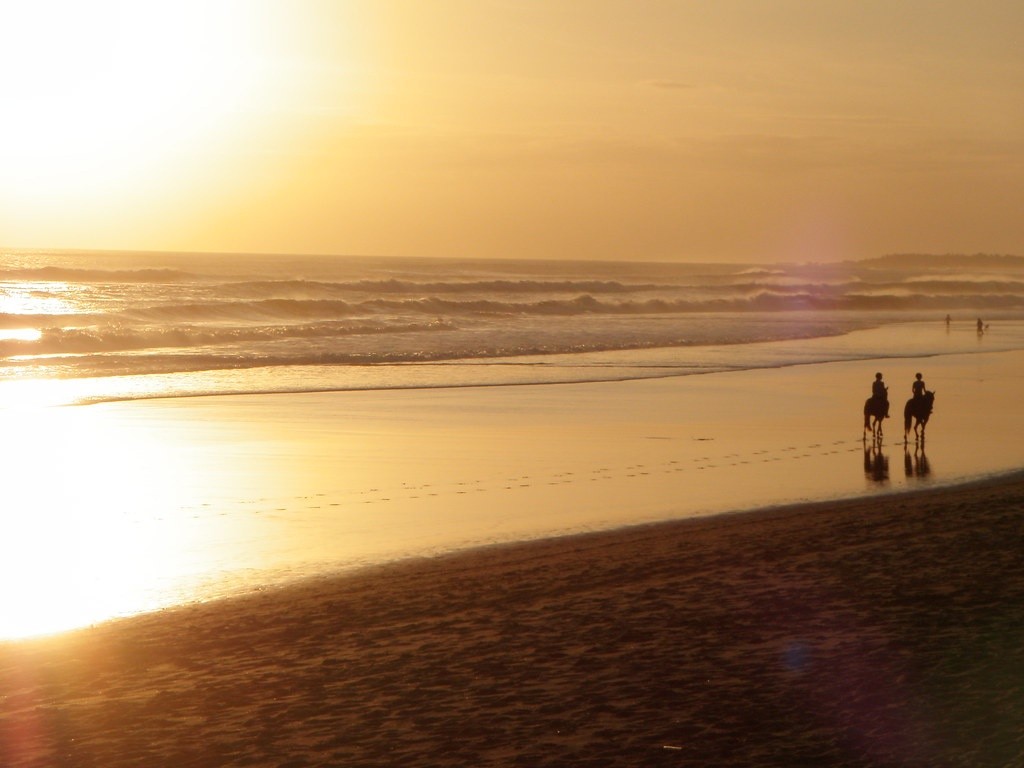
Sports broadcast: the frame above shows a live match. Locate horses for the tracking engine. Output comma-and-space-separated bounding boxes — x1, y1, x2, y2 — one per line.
862, 387, 890, 437
904, 390, 935, 440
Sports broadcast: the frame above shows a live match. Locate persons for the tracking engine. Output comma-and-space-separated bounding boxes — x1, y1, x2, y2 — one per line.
912, 373, 926, 399
945, 314, 952, 326
976, 318, 984, 333
872, 373, 890, 418
